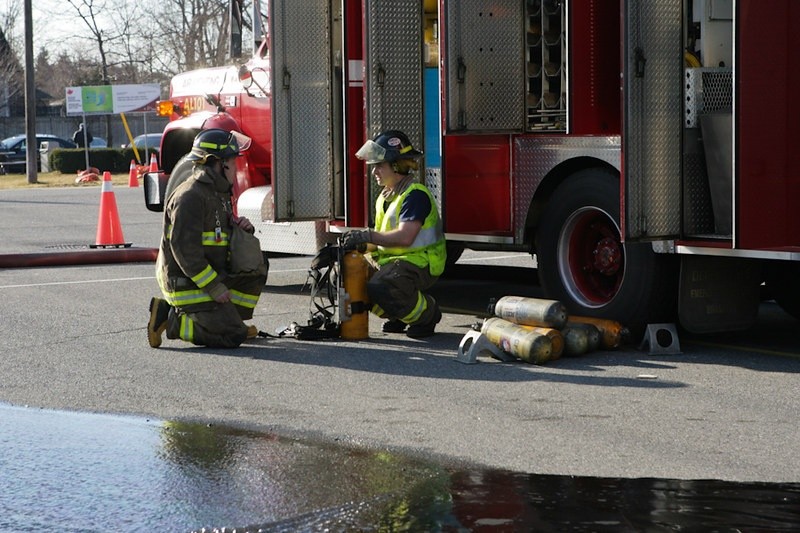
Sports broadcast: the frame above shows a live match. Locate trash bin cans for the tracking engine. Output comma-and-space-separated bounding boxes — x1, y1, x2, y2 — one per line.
38, 141, 61, 172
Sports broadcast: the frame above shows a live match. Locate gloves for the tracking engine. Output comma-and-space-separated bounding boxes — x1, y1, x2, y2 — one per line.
342, 227, 373, 246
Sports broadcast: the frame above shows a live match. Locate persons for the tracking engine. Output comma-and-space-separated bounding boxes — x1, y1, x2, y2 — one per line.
145, 127, 269, 351
329, 130, 447, 339
73, 124, 93, 148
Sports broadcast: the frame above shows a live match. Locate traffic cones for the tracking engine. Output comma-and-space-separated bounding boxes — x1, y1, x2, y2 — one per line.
128, 159, 140, 187
86, 171, 133, 249
148, 151, 159, 174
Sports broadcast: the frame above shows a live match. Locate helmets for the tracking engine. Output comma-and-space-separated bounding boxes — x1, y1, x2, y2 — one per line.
185, 129, 244, 161
365, 131, 423, 164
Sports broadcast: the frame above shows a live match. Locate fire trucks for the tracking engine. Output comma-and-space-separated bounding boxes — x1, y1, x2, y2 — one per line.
136, 0, 800, 346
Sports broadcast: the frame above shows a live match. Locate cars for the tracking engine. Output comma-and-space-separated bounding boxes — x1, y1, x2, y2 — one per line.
0, 132, 77, 176
88, 136, 107, 148
120, 132, 164, 149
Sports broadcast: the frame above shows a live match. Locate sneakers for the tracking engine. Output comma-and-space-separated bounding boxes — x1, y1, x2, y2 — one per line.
407, 302, 442, 337
246, 323, 258, 339
382, 317, 407, 332
148, 297, 171, 347
